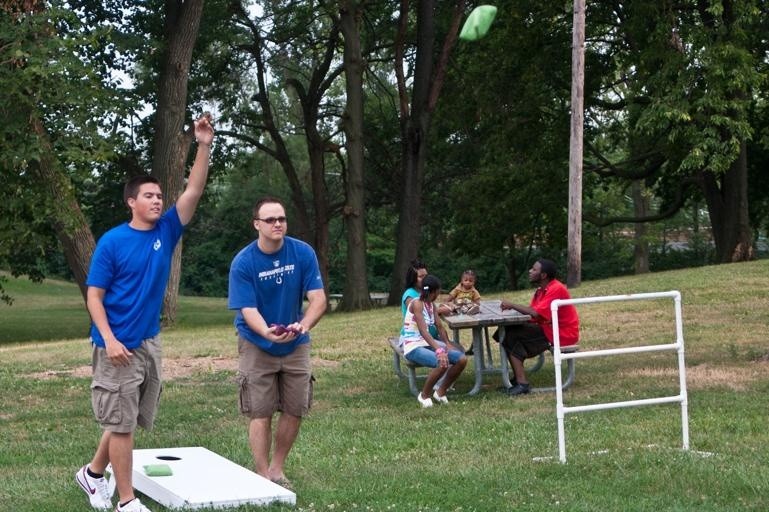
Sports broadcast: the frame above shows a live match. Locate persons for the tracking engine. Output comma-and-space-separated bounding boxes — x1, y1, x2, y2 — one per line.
403, 275, 468, 407
493, 259, 579, 396
74, 116, 215, 512
399, 259, 465, 392
228, 198, 327, 484
439, 269, 481, 316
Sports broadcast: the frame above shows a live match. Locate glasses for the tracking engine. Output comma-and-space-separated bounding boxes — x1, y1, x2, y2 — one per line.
254, 217, 286, 223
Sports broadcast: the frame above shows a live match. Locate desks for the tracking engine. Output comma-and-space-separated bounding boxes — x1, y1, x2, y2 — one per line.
435, 299, 532, 400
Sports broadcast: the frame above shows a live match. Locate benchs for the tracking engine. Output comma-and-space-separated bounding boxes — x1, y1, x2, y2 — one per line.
387, 337, 464, 397
532, 343, 581, 394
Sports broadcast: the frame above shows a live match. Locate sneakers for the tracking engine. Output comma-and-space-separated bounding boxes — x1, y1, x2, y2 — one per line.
498, 379, 529, 394
418, 392, 432, 406
433, 391, 448, 403
76, 464, 113, 508
116, 498, 152, 512
